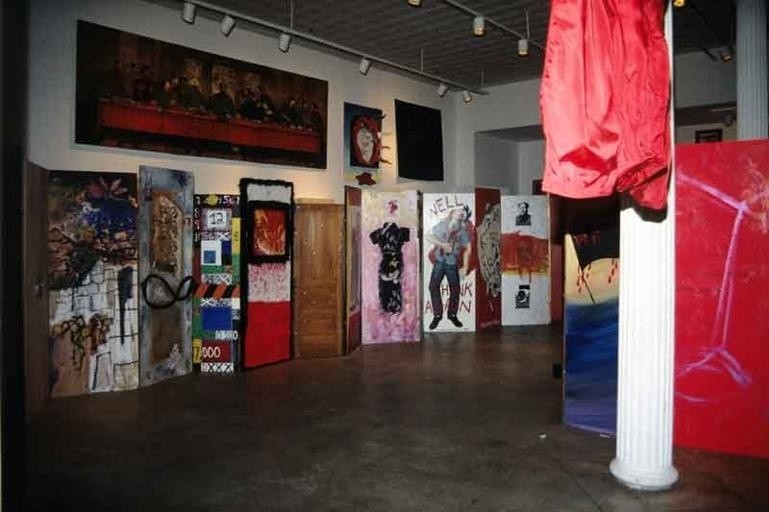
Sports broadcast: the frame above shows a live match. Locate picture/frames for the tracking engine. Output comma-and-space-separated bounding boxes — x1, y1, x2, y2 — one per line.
340, 100, 384, 190
69, 18, 329, 174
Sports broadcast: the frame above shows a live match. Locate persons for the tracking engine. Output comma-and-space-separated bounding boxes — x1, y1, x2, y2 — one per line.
97, 67, 322, 133
515, 202, 530, 225
422, 208, 472, 329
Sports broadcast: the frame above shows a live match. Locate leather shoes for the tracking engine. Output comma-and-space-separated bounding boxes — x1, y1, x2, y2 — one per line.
450, 316, 463, 327
430, 317, 442, 328
